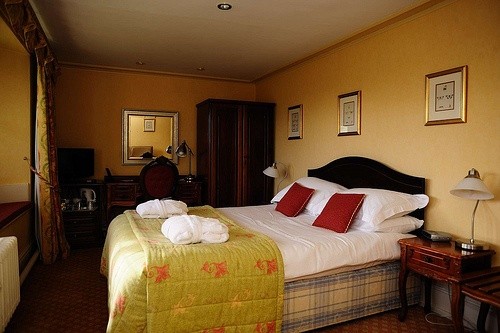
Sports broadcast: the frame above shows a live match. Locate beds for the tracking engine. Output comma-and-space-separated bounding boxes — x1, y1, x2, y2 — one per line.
101, 157, 428, 333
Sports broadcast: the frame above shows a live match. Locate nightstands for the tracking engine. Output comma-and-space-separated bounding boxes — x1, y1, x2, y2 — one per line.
397, 235, 496, 333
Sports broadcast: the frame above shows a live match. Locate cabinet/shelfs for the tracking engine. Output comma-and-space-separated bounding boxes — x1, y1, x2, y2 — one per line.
195, 99, 277, 209
104, 175, 201, 210
61, 205, 101, 247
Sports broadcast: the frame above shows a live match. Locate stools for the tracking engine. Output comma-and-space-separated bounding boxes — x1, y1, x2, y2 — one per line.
448, 266, 500, 333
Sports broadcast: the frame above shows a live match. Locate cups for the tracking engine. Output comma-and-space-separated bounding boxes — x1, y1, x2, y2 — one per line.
61, 197, 95, 210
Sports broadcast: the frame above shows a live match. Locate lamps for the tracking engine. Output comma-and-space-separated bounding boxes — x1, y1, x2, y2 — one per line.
175, 139, 195, 184
262, 162, 288, 195
166, 146, 171, 154
449, 169, 495, 250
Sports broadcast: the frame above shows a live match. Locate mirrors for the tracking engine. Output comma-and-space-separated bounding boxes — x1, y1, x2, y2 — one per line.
122, 110, 178, 166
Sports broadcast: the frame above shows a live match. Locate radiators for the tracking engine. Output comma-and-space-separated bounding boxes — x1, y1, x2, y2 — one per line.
0, 236, 21, 333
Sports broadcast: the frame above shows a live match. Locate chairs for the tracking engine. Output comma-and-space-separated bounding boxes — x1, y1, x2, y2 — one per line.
136, 155, 180, 206
140, 152, 155, 157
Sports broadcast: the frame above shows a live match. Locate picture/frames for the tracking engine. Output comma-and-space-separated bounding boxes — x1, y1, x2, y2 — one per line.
287, 103, 304, 140
336, 90, 361, 136
424, 65, 468, 126
144, 119, 156, 132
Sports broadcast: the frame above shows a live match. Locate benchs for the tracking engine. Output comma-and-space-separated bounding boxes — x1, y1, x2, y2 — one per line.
131, 146, 153, 157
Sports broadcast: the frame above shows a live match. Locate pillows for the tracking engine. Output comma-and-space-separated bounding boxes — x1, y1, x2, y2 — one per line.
271, 176, 429, 233
